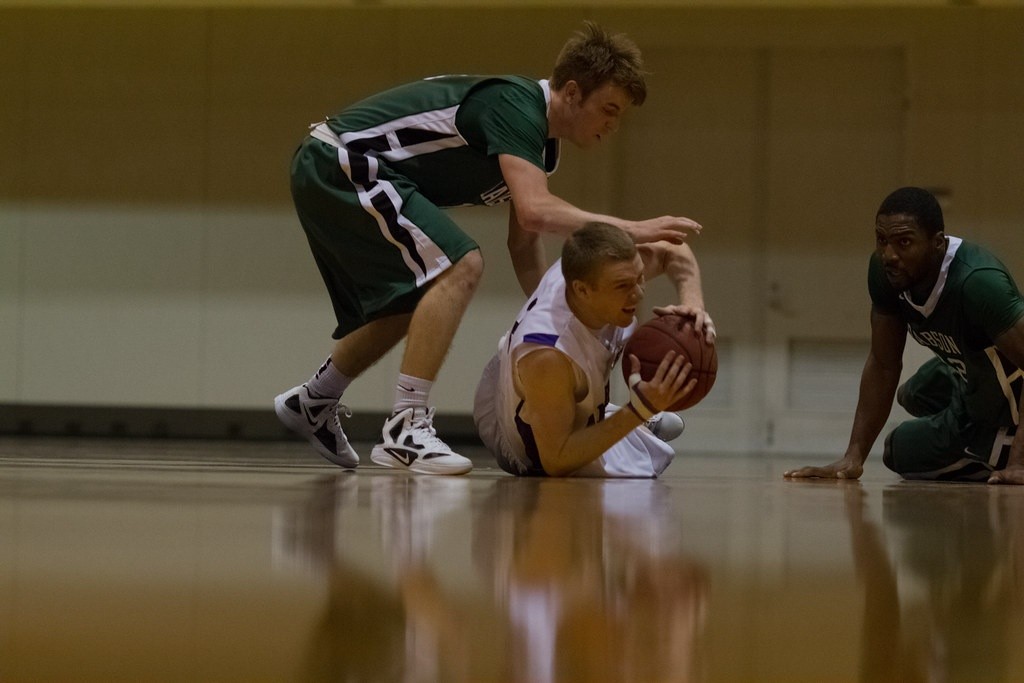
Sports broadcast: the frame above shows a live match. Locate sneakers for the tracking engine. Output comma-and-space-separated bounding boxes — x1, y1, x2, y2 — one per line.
274, 383, 360, 467
641, 410, 685, 442
371, 407, 473, 477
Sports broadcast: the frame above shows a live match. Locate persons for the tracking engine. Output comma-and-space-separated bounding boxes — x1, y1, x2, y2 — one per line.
273, 21, 716, 478
279, 474, 1024, 683
783, 187, 1024, 485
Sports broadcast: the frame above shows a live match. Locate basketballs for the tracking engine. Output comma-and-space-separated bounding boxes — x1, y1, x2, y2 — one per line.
622, 315, 719, 412
